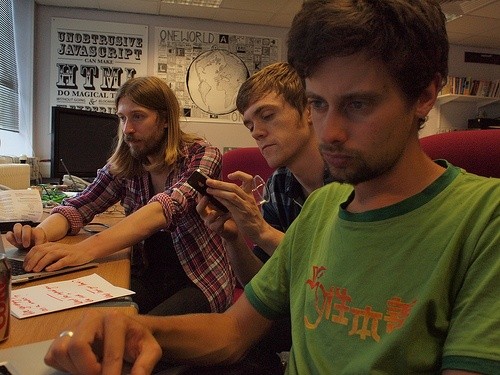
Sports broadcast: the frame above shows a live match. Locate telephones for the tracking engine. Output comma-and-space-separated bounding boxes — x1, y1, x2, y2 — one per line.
63, 174, 92, 191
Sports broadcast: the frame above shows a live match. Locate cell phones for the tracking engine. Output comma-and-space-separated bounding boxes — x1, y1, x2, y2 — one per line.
187, 169, 229, 214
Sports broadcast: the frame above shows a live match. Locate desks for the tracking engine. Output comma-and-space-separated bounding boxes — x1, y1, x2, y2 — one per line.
0, 182, 131, 347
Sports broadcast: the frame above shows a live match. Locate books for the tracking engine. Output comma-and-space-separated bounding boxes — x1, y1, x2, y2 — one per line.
439, 76, 499, 97
5, 244, 100, 285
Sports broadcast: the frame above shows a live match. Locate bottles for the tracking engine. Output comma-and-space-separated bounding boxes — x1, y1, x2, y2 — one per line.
0, 234, 13, 344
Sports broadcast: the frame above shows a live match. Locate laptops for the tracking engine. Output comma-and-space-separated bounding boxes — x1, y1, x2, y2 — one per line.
0, 230, 99, 286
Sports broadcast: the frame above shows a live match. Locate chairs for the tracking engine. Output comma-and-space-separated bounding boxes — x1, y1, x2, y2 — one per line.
418, 127, 499, 179
184, 147, 292, 375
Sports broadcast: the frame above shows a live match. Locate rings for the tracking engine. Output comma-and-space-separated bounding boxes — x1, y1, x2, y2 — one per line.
60, 330, 74, 336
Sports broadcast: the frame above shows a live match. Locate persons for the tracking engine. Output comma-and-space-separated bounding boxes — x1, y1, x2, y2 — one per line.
42, 0, 500, 375
5, 77, 232, 319
194, 60, 334, 375
